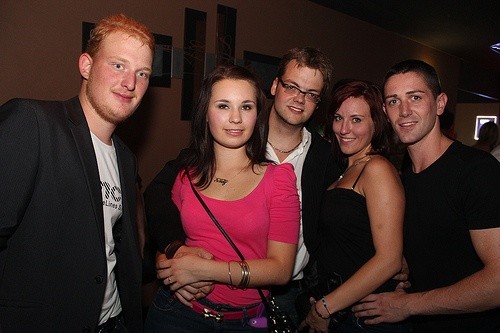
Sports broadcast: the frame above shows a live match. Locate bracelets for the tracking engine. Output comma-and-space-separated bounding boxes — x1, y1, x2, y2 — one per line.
314, 295, 332, 320
227, 260, 251, 289
165, 236, 187, 258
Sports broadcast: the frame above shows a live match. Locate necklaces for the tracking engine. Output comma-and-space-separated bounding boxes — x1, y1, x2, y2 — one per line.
265, 131, 303, 152
209, 159, 251, 186
337, 155, 367, 182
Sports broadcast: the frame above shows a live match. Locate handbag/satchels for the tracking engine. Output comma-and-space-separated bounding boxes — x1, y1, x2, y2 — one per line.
262, 281, 308, 333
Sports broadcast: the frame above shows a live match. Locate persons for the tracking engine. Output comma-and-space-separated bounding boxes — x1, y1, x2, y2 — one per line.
350, 59, 500, 333
301, 79, 406, 333
470, 120, 500, 162
152, 63, 301, 333
0, 11, 215, 333
143, 147, 198, 237
258, 47, 411, 333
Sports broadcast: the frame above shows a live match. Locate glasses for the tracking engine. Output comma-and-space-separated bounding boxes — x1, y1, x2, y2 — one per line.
278, 75, 322, 103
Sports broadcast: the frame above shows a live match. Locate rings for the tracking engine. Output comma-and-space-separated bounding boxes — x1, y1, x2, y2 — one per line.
168, 276, 172, 284
